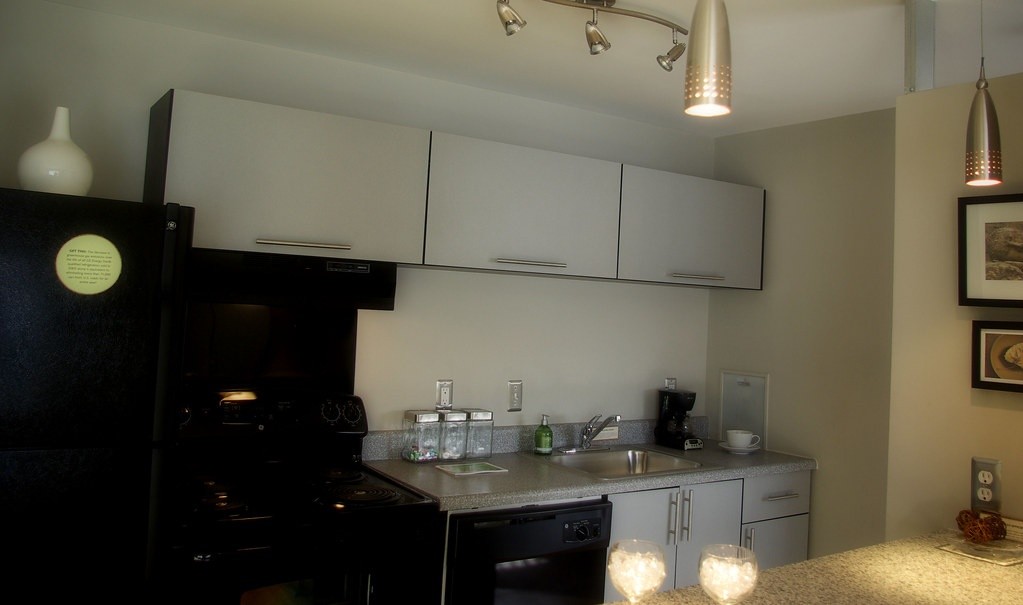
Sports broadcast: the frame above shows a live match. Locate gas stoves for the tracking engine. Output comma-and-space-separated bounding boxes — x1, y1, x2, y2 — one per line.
164, 387, 439, 551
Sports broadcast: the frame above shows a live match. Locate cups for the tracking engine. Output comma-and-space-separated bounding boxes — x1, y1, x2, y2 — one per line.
699, 544, 760, 605
727, 430, 760, 447
608, 540, 666, 605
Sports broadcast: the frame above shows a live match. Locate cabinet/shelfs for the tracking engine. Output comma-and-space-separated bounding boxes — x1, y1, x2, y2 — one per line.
603, 469, 812, 601
142, 89, 767, 292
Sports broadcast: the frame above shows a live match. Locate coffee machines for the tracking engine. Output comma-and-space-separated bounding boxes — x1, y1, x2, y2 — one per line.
654, 378, 704, 450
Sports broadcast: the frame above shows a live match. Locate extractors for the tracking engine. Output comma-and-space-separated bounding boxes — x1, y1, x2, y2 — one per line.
178, 246, 398, 310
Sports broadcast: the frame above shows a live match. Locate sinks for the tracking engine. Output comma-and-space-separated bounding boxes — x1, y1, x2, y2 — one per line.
544, 447, 724, 480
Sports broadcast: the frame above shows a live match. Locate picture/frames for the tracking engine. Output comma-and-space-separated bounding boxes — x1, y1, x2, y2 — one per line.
956, 194, 1023, 307
968, 319, 1023, 393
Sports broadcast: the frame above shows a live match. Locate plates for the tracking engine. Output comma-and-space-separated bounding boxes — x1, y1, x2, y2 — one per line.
718, 442, 760, 454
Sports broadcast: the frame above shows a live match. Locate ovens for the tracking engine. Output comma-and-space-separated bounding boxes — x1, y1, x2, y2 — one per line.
174, 543, 440, 605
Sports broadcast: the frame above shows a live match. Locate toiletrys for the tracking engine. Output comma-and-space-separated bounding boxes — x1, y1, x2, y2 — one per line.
533, 413, 553, 455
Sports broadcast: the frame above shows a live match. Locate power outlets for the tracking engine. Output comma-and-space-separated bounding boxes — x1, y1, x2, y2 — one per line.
508, 382, 523, 413
969, 457, 1002, 519
435, 379, 454, 409
664, 378, 676, 391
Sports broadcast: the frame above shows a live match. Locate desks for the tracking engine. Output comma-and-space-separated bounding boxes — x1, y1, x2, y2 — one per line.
604, 519, 1023, 605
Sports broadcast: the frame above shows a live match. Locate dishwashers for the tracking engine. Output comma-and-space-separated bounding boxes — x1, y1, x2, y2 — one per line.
445, 499, 612, 605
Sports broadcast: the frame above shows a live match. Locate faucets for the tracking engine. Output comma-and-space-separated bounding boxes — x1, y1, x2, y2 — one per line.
578, 413, 622, 449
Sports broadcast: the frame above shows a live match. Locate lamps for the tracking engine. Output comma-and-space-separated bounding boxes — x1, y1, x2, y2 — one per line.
580, 9, 611, 56
683, 0, 732, 118
496, 1, 526, 37
657, 26, 687, 73
965, 0, 1004, 189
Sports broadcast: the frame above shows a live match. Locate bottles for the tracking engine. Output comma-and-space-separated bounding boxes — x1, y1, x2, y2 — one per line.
436, 410, 467, 460
17, 107, 93, 196
400, 410, 440, 462
460, 408, 494, 459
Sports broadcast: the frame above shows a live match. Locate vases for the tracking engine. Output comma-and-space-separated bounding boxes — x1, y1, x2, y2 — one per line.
15, 106, 94, 197
